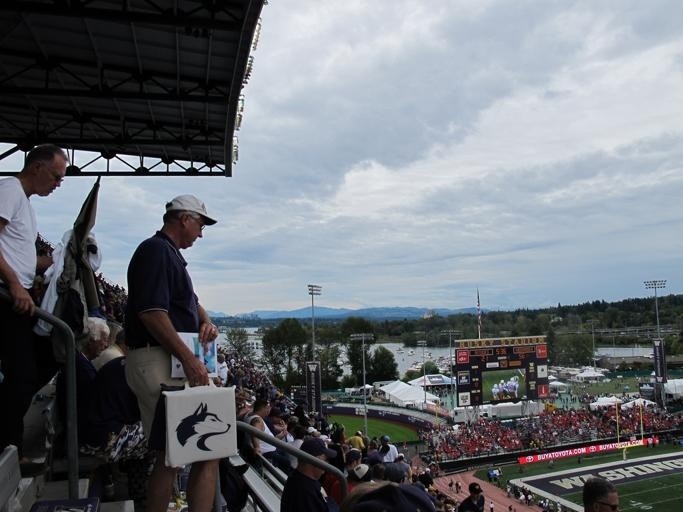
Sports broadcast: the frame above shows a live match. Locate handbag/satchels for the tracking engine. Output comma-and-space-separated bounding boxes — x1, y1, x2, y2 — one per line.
161, 385, 239, 468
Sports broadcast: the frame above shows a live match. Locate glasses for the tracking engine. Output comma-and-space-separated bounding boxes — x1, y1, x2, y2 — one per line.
598, 502, 619, 512
53, 174, 64, 183
190, 216, 205, 230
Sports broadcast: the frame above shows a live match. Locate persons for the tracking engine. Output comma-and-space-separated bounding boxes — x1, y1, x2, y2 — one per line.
56, 317, 187, 503
125, 194, 219, 512
430, 393, 683, 460
282, 412, 439, 511
582, 478, 619, 511
220, 350, 309, 470
492, 376, 519, 401
1, 144, 67, 461
438, 469, 561, 511
567, 375, 650, 393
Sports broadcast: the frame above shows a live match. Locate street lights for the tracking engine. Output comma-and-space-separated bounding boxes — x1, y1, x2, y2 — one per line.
307, 284, 322, 362
642, 279, 668, 338
440, 329, 464, 409
350, 332, 374, 436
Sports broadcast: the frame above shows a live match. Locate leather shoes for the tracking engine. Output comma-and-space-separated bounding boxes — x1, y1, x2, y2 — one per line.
20, 461, 50, 477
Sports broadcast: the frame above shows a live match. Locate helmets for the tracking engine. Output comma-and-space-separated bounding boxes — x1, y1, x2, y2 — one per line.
346, 449, 361, 464
350, 463, 374, 481
300, 438, 338, 458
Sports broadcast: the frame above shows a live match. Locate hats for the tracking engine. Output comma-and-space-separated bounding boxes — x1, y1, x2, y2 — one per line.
470, 483, 483, 494
165, 195, 217, 225
398, 453, 404, 457
379, 436, 389, 442
339, 480, 437, 512
